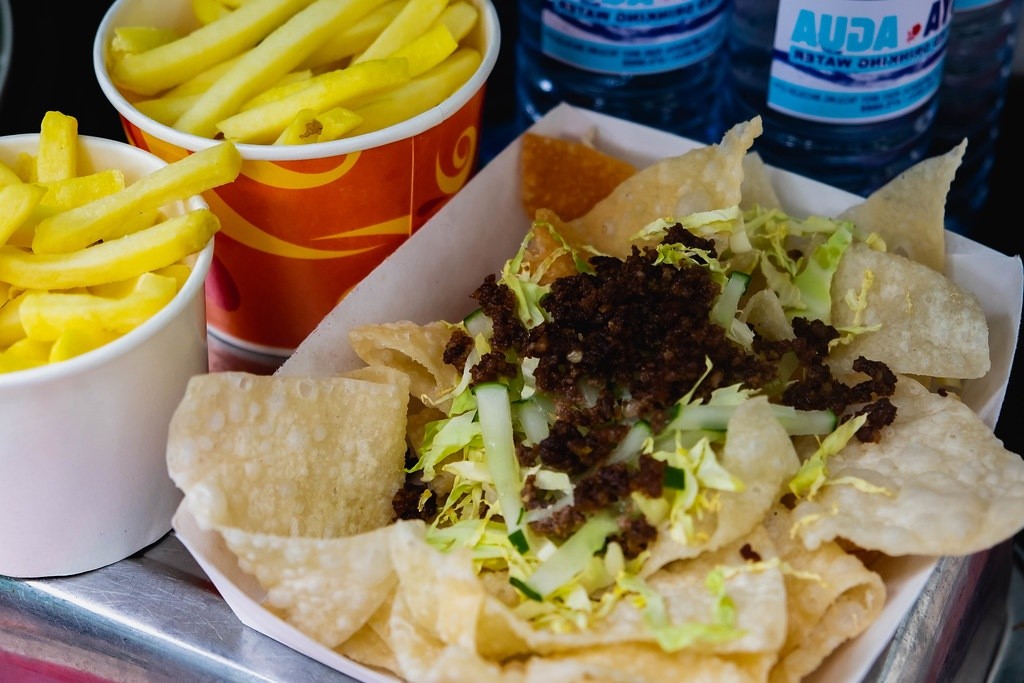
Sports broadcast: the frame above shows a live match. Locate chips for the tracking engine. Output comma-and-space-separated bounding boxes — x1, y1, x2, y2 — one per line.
162, 116, 1024, 683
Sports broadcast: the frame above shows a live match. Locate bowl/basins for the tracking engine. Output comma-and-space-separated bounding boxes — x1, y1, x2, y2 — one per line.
0, 132, 213, 578
93, 0, 503, 354
173, 98, 1023, 683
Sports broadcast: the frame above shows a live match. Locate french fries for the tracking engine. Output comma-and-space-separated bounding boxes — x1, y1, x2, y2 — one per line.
0, 109, 242, 375
108, 0, 480, 148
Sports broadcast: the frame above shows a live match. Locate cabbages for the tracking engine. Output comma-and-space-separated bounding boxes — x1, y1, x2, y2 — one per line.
396, 193, 916, 641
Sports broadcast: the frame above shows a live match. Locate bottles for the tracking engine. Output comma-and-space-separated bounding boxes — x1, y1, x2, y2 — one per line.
944, 0, 1020, 220
514, 0, 734, 145
720, 0, 956, 200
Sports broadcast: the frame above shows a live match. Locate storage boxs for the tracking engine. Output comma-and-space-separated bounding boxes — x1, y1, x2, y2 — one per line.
173, 100, 1024, 683
